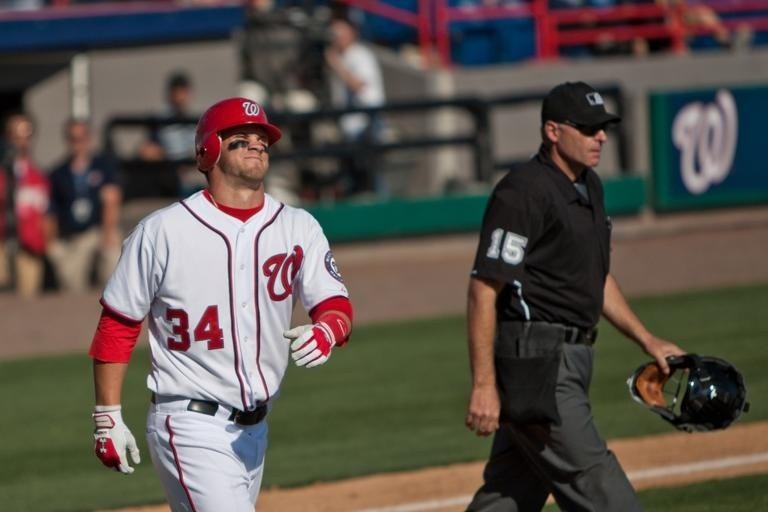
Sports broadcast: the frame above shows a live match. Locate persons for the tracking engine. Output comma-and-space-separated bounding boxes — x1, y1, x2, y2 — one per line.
88, 97, 354, 512
465, 82, 689, 512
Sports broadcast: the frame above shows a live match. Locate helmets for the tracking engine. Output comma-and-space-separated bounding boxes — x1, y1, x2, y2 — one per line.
625, 350, 752, 434
194, 97, 285, 173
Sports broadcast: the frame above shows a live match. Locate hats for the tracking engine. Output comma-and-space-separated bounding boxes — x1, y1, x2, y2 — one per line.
541, 81, 623, 131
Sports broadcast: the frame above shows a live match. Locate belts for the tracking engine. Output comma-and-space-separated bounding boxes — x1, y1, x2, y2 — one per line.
151, 390, 269, 427
562, 325, 600, 346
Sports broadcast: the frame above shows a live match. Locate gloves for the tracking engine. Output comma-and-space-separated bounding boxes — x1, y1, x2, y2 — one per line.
282, 320, 337, 369
89, 403, 141, 476
